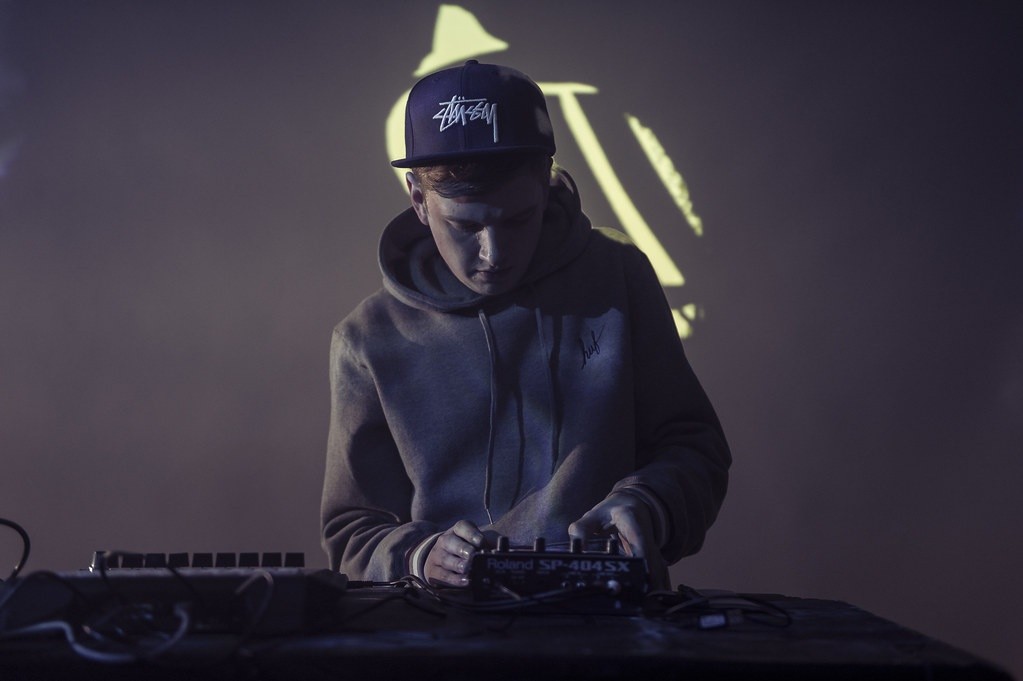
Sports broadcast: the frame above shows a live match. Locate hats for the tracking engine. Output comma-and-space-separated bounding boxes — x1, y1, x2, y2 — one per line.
391, 60, 557, 168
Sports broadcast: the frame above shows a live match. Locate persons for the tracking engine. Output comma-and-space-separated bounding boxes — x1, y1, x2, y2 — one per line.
318, 57, 733, 590
